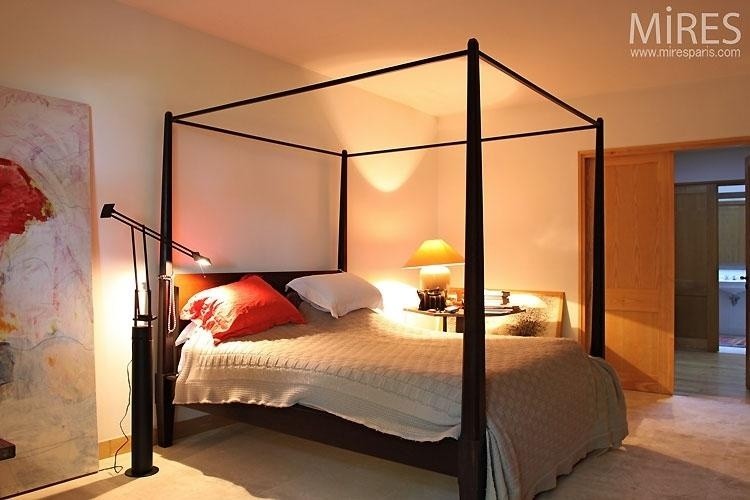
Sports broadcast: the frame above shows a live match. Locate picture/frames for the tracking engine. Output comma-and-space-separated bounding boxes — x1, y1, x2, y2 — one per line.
486, 289, 565, 341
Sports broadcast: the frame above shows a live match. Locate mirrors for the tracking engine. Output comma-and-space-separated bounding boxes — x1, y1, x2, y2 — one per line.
718, 201, 746, 270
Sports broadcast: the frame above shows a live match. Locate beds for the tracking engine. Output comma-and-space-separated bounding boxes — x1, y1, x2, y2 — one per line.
155, 37, 605, 500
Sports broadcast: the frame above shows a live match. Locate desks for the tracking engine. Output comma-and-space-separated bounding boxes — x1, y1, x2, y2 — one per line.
405, 308, 524, 332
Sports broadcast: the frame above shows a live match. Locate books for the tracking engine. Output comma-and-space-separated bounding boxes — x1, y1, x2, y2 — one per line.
484, 305, 519, 315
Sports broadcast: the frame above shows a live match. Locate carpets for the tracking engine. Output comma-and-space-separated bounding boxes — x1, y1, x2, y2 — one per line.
719, 335, 746, 348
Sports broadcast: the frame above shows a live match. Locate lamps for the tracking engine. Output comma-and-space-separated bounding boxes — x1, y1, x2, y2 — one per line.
401, 238, 465, 293
101, 203, 211, 477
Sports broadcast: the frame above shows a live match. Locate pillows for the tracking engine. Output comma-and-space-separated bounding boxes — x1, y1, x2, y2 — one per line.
180, 274, 306, 346
285, 272, 383, 318
